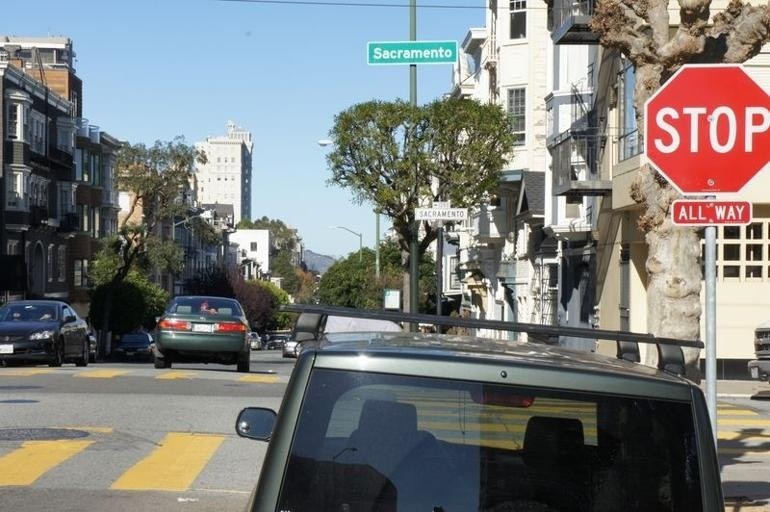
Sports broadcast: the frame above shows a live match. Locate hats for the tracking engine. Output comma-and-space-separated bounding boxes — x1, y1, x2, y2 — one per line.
86, 326, 98, 363
153, 297, 260, 373
119, 326, 321, 359
0, 300, 91, 367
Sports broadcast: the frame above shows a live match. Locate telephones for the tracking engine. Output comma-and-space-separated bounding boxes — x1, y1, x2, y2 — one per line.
364, 38, 460, 65
412, 205, 467, 221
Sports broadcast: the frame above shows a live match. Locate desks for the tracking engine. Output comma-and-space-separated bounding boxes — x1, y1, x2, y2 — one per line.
328, 224, 363, 264
314, 138, 381, 282
171, 209, 212, 295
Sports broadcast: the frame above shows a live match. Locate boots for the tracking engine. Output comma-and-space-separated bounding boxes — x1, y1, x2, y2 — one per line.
231, 301, 724, 512
746, 318, 770, 385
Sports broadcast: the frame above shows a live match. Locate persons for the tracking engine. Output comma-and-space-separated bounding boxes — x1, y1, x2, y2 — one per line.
12, 310, 22, 320
39, 311, 54, 320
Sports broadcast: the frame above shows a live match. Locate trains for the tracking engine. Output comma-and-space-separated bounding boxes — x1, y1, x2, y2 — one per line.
643, 61, 770, 196
